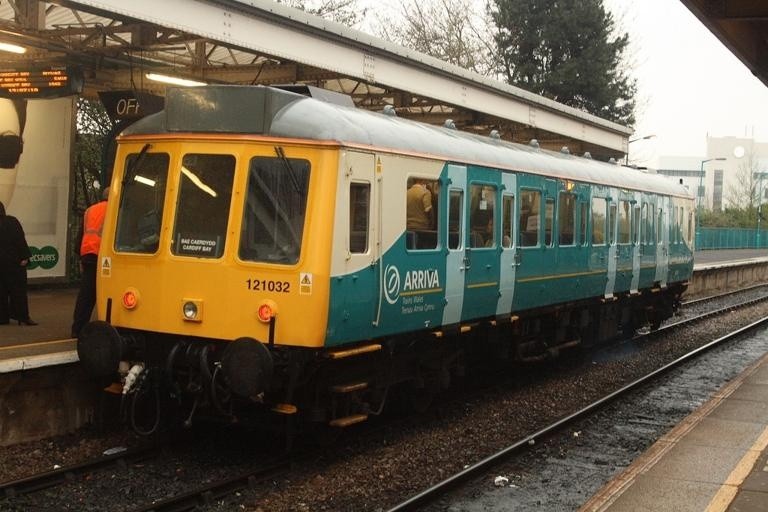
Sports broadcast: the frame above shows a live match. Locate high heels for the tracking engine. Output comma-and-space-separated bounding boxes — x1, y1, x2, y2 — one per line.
18, 316, 38, 326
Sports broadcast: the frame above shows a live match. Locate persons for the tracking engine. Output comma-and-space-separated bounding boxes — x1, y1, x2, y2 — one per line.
1, 202, 39, 327
484, 218, 510, 247
70, 186, 110, 337
407, 179, 438, 230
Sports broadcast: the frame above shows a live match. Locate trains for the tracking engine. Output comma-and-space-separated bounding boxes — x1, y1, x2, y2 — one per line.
77, 84, 695, 452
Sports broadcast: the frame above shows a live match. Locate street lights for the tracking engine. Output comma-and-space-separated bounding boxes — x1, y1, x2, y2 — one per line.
697, 158, 726, 249
626, 134, 656, 167
